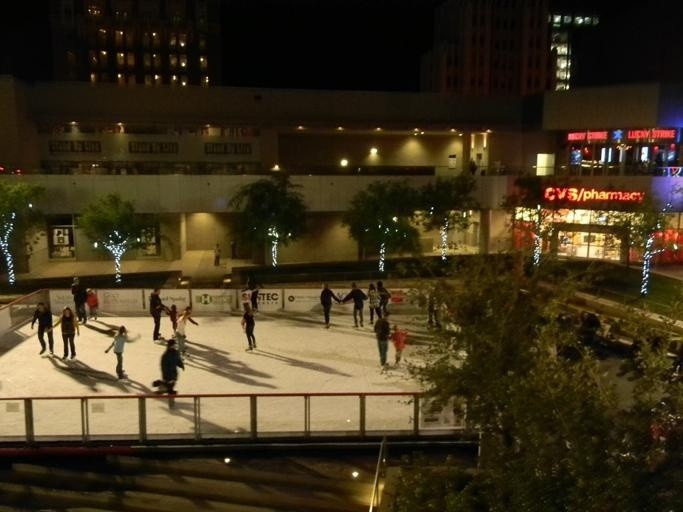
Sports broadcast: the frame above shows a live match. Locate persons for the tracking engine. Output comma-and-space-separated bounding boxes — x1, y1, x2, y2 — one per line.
69, 276, 86, 323
85, 288, 99, 320
243, 271, 259, 312
32, 302, 53, 355
175, 310, 190, 358
230, 240, 236, 258
179, 306, 198, 326
240, 302, 256, 350
241, 287, 258, 313
318, 280, 443, 371
105, 326, 140, 379
214, 243, 220, 265
150, 288, 167, 341
53, 307, 80, 360
152, 339, 185, 394
166, 303, 177, 339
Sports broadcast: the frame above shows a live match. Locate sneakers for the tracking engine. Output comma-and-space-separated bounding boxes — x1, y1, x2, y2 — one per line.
154, 333, 164, 340
39, 347, 46, 354
62, 353, 68, 359
70, 352, 76, 360
